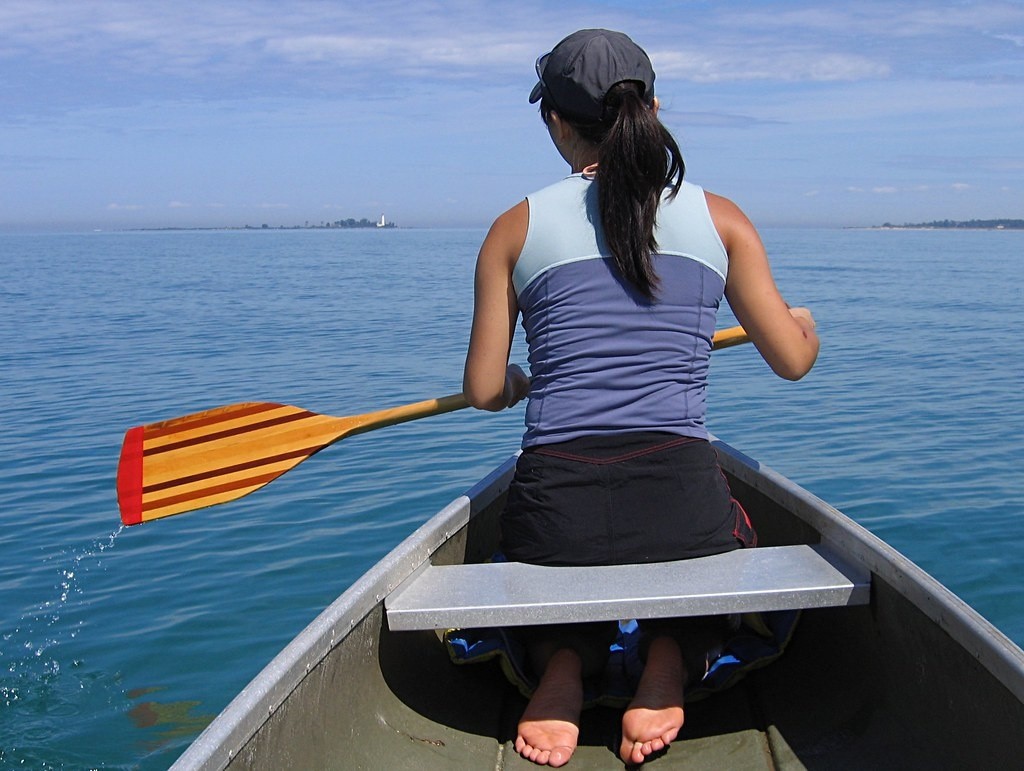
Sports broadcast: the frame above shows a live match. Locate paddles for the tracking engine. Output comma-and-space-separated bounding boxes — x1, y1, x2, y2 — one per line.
115, 298, 793, 527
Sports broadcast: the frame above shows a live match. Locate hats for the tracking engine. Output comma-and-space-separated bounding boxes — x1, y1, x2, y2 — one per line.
528, 29, 655, 128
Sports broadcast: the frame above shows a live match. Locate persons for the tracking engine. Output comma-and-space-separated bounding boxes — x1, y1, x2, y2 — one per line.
462, 30, 819, 765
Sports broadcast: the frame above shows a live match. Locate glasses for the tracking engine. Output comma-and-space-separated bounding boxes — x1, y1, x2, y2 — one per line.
535, 50, 561, 116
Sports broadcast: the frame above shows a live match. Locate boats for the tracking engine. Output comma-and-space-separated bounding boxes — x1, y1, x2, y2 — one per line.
166, 431, 1024, 771
